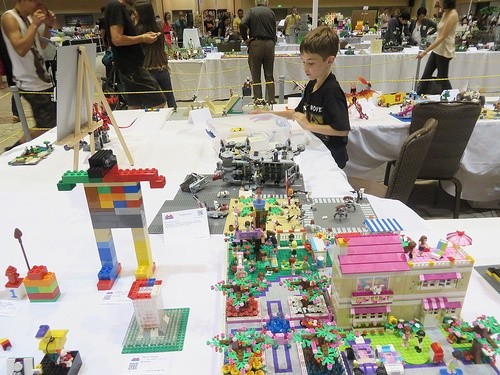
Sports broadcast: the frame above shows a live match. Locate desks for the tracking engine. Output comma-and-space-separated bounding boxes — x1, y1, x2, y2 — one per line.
0, 25, 500, 375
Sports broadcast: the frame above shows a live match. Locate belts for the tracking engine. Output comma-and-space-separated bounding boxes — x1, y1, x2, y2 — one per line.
253, 37, 272, 40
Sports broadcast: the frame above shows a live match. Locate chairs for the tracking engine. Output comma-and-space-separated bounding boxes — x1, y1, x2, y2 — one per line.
384, 101, 481, 219
385, 118, 439, 205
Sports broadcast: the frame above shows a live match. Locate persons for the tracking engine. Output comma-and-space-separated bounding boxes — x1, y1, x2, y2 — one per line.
381, 8, 389, 24
157, 11, 186, 48
416, 0, 458, 89
0, 11, 21, 123
134, 2, 177, 108
239, 0, 278, 105
76, 19, 81, 27
277, 18, 285, 32
250, 25, 350, 167
284, 7, 301, 36
386, 12, 409, 42
308, 14, 320, 26
94, 20, 101, 34
409, 6, 437, 43
1, 0, 57, 128
204, 8, 245, 38
104, 0, 168, 109
458, 14, 477, 29
99, 7, 110, 50
432, 14, 436, 21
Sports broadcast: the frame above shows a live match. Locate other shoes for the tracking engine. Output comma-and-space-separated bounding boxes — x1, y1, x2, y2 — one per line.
13, 115, 20, 123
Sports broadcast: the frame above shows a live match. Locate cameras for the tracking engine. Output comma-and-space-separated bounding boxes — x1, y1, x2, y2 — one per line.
180, 173, 210, 192
